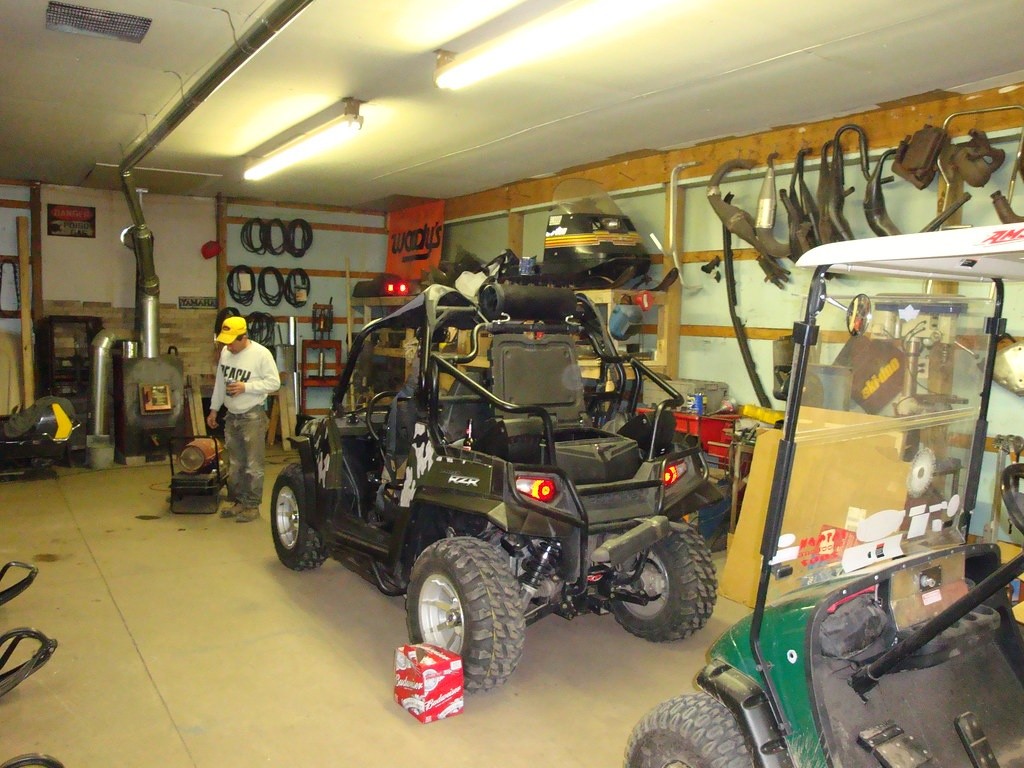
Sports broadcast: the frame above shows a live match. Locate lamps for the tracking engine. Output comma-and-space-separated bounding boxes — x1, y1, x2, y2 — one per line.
243, 99, 365, 182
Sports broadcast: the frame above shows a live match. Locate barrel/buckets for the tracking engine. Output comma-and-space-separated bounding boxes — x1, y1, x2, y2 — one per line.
687, 395, 707, 415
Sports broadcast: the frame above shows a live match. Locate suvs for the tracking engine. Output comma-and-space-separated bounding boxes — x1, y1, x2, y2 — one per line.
266, 286, 721, 698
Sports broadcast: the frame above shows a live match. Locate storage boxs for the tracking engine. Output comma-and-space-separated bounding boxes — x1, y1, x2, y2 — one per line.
393, 642, 464, 723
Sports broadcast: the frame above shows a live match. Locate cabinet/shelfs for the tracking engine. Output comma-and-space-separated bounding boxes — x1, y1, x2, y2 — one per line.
352, 295, 492, 389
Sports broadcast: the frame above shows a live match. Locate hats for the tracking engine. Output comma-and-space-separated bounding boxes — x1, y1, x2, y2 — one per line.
216, 317, 247, 345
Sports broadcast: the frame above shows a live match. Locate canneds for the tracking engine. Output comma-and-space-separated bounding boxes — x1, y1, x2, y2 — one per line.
226, 378, 237, 396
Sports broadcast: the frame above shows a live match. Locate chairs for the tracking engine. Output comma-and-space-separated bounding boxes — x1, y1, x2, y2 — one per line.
438, 369, 484, 440
301, 338, 341, 415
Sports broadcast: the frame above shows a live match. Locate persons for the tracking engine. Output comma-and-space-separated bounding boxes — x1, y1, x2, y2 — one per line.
207, 316, 281, 523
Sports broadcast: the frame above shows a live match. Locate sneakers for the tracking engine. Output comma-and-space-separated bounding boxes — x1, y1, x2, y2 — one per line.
221, 502, 244, 517
236, 507, 260, 522
